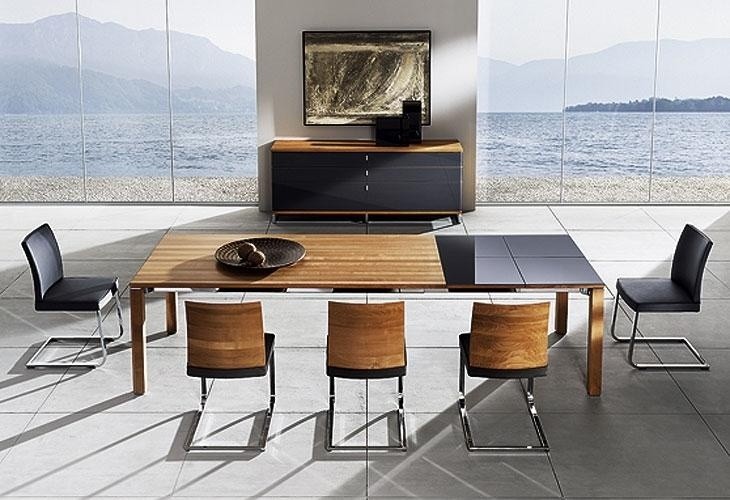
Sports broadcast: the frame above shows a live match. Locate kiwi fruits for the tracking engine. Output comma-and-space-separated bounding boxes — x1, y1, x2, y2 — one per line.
236, 240, 266, 264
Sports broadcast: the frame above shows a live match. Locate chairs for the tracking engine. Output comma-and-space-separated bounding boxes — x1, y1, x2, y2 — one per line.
612, 224, 715, 372
21, 222, 124, 370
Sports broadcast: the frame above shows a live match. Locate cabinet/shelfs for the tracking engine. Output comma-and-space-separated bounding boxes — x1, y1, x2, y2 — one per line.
268, 139, 464, 225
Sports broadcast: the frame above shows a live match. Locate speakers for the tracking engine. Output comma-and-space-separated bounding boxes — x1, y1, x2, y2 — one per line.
402, 100, 422, 144
376, 117, 407, 145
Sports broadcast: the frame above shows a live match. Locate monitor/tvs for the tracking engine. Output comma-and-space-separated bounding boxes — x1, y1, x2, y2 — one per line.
302, 30, 431, 126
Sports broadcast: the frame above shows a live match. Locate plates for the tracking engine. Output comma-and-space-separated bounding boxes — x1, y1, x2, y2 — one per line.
214, 237, 308, 271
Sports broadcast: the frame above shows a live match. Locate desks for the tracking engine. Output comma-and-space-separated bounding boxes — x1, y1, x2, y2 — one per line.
129, 229, 605, 398
